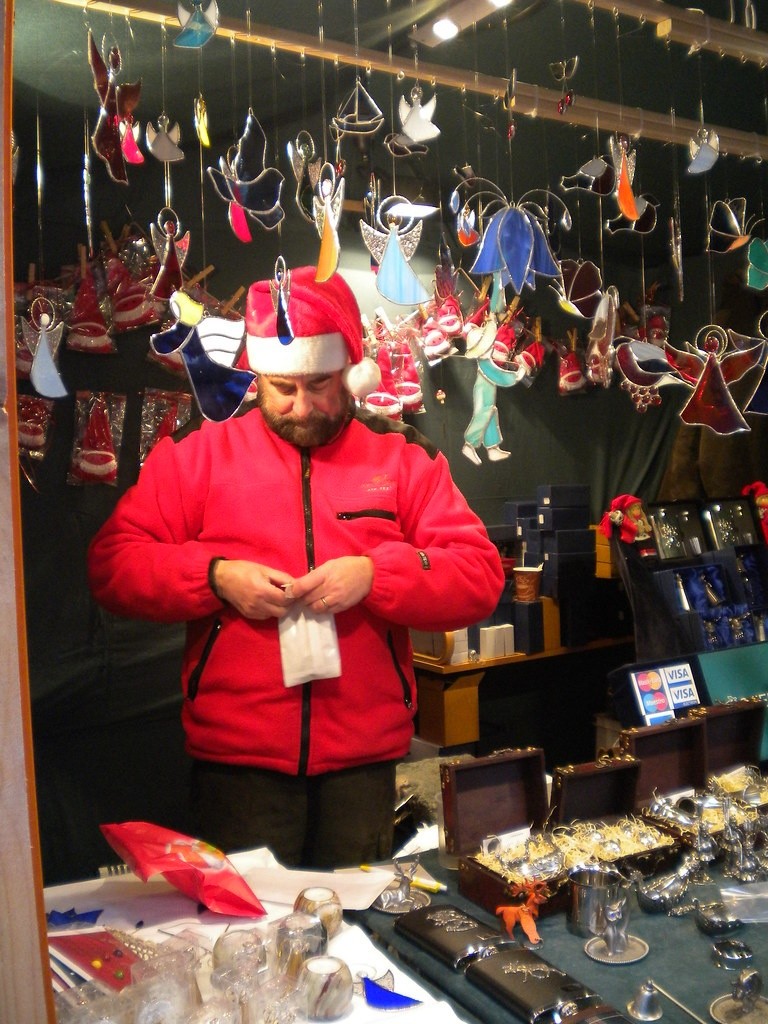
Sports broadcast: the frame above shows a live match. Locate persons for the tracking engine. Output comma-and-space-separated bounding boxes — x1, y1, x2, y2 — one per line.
611, 495, 652, 543
87, 266, 507, 872
742, 482, 768, 520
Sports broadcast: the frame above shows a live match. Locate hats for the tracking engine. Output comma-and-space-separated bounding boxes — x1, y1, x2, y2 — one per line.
245, 265, 381, 398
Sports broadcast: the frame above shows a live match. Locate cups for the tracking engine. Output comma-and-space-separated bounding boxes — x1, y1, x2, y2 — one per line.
210, 930, 267, 990
294, 887, 342, 941
296, 956, 353, 1021
567, 869, 620, 938
513, 567, 543, 603
276, 912, 328, 973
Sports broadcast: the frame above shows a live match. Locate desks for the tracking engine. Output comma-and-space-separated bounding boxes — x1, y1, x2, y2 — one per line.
406, 632, 632, 746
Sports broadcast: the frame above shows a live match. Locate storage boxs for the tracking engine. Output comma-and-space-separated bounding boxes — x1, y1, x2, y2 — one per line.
409, 484, 623, 663
437, 746, 571, 926
550, 752, 683, 886
617, 718, 759, 863
688, 693, 768, 817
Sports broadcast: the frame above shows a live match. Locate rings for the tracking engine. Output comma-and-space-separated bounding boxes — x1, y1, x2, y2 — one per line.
322, 597, 328, 608
